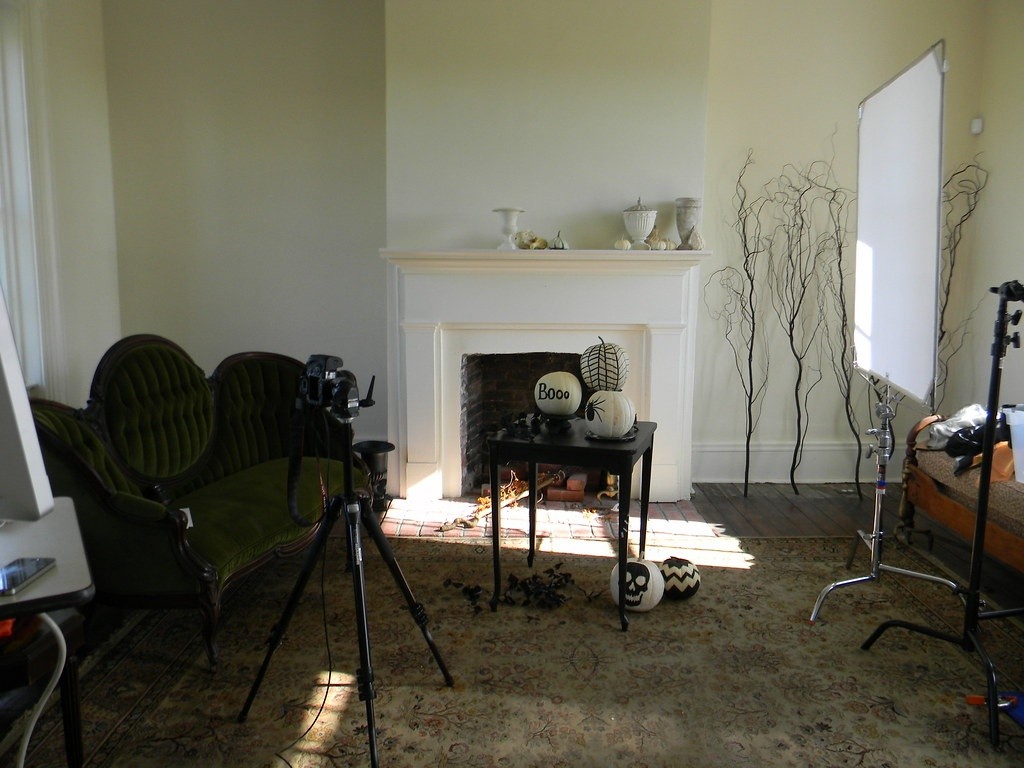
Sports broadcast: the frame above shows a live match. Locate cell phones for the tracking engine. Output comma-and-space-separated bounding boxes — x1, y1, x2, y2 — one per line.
0, 558, 56, 597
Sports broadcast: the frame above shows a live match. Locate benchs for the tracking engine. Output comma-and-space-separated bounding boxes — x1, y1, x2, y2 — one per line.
27, 333, 373, 674
893, 413, 1023, 573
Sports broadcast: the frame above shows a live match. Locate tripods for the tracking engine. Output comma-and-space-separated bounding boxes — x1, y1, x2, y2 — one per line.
239, 417, 456, 768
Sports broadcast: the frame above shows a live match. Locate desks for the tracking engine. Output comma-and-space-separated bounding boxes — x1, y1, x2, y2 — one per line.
486, 416, 657, 630
1, 494, 97, 768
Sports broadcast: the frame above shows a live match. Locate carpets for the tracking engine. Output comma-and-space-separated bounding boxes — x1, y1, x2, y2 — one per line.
1, 533, 1023, 768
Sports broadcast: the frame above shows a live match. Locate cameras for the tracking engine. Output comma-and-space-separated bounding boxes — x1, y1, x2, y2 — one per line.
303, 354, 361, 417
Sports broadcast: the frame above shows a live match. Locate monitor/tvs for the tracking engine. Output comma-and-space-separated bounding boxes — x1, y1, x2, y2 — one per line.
0, 285, 53, 530
